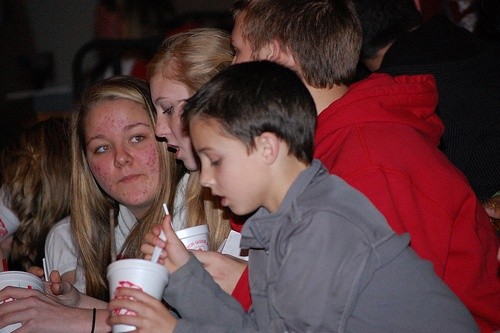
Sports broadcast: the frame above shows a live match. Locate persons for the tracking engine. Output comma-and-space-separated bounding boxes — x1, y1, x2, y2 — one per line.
354, 0, 500, 201
107, 59, 478, 333
186, 0, 499, 333
0, 0, 254, 333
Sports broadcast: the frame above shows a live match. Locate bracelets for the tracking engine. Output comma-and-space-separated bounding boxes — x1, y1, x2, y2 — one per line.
91, 308, 96, 333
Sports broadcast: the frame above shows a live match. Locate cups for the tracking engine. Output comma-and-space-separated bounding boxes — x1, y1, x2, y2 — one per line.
0, 271, 45, 333
0, 203, 21, 243
167, 225, 211, 255
106, 258, 169, 333
